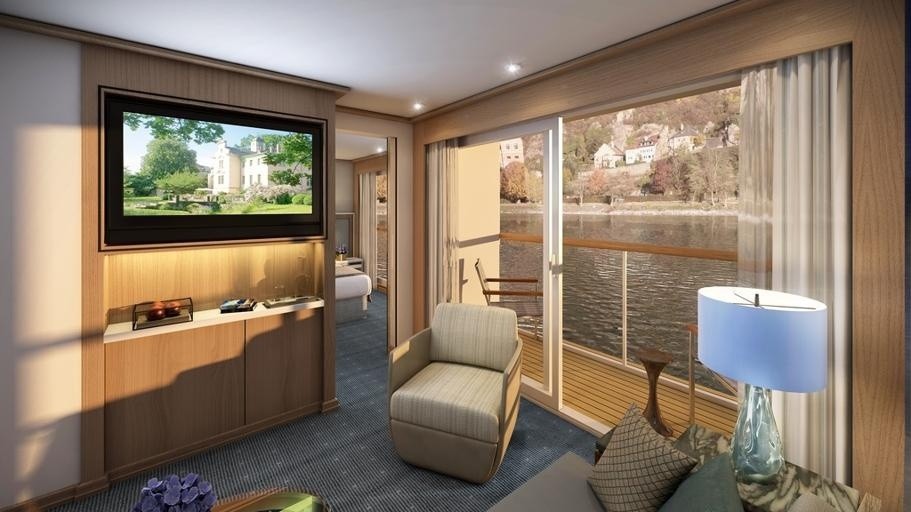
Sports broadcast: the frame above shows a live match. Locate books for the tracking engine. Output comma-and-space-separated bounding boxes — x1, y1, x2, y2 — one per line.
221, 302, 257, 313
220, 297, 255, 310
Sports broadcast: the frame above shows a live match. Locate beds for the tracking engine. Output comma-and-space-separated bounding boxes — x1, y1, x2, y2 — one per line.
333, 261, 372, 325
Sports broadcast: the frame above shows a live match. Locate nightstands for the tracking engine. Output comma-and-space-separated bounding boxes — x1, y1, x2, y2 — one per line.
346, 256, 364, 272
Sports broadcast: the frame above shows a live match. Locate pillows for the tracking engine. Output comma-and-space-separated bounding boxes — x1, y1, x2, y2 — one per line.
585, 402, 697, 512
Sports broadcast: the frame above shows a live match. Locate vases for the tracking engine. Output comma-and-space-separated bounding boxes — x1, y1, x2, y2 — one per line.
336, 254, 344, 261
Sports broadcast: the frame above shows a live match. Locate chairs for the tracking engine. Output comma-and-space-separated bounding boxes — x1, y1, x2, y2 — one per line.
475, 259, 543, 343
388, 301, 524, 484
481, 422, 882, 512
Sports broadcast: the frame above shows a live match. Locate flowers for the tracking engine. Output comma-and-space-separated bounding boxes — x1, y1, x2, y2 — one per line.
335, 242, 351, 256
130, 470, 219, 512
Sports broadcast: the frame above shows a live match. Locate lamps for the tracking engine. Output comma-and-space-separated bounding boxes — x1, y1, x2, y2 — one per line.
697, 285, 830, 486
